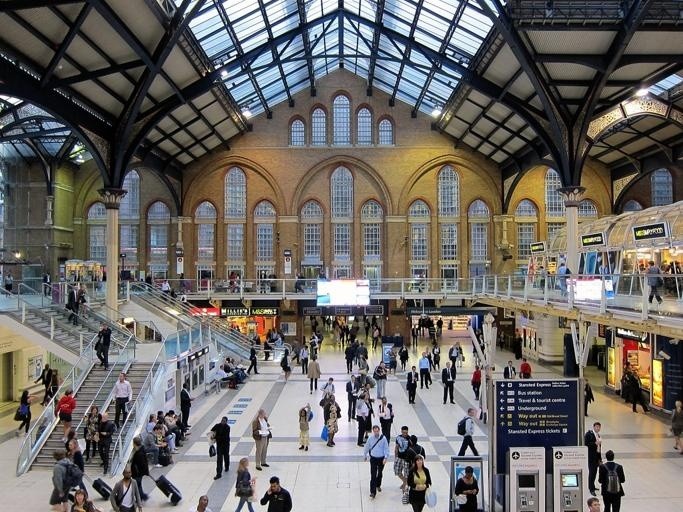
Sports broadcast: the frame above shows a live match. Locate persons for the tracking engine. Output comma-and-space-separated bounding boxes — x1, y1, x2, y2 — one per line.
5, 272, 13, 297
190, 496, 212, 512
583, 361, 683, 512
145, 273, 186, 304
95, 324, 111, 370
229, 271, 240, 293
538, 260, 682, 303
43, 272, 86, 325
15, 365, 194, 512
259, 271, 327, 294
208, 316, 532, 512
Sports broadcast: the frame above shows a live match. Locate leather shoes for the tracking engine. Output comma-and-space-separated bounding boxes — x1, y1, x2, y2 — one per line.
103, 470, 107, 474
255, 467, 261, 471
377, 487, 381, 492
224, 468, 228, 472
100, 460, 104, 467
351, 417, 356, 419
590, 490, 595, 496
213, 474, 221, 480
298, 445, 303, 449
593, 488, 599, 490
114, 423, 119, 428
304, 446, 307, 451
425, 385, 429, 390
260, 464, 269, 467
369, 490, 374, 498
443, 401, 445, 404
420, 386, 422, 390
409, 401, 412, 403
412, 402, 415, 403
449, 400, 455, 404
348, 418, 350, 421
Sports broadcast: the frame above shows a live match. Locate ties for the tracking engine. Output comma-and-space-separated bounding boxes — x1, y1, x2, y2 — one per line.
510, 366, 511, 378
381, 406, 383, 412
412, 373, 413, 384
447, 369, 449, 379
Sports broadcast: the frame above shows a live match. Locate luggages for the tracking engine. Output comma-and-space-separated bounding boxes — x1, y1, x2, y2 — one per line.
147, 474, 181, 506
83, 474, 112, 500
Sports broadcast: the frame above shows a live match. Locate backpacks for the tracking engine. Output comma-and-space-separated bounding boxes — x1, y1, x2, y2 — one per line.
55, 460, 82, 486
602, 464, 621, 495
409, 446, 423, 465
280, 356, 287, 367
59, 398, 72, 414
457, 416, 471, 435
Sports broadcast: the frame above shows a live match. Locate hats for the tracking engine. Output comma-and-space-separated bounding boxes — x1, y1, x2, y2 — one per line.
52, 449, 66, 460
102, 322, 106, 325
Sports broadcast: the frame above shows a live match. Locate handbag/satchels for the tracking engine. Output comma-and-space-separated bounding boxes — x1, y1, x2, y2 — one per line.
455, 495, 467, 506
234, 483, 253, 498
424, 489, 436, 508
208, 445, 215, 457
94, 342, 98, 350
13, 411, 25, 421
406, 383, 409, 391
306, 411, 312, 422
167, 455, 173, 464
86, 503, 100, 512
320, 426, 327, 442
124, 402, 134, 413
368, 449, 370, 456
84, 427, 88, 438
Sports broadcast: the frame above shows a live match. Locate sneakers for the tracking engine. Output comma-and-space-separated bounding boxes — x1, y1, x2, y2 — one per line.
331, 442, 334, 445
362, 441, 365, 444
14, 429, 19, 437
376, 397, 382, 399
63, 437, 67, 443
84, 456, 88, 462
39, 401, 43, 404
173, 447, 178, 450
186, 425, 191, 429
179, 437, 187, 441
364, 436, 368, 438
153, 464, 163, 467
104, 367, 107, 371
326, 443, 333, 447
62, 437, 64, 441
100, 362, 104, 366
23, 433, 28, 438
170, 450, 179, 454
92, 451, 95, 457
185, 428, 189, 431
176, 444, 182, 447
357, 444, 363, 446
